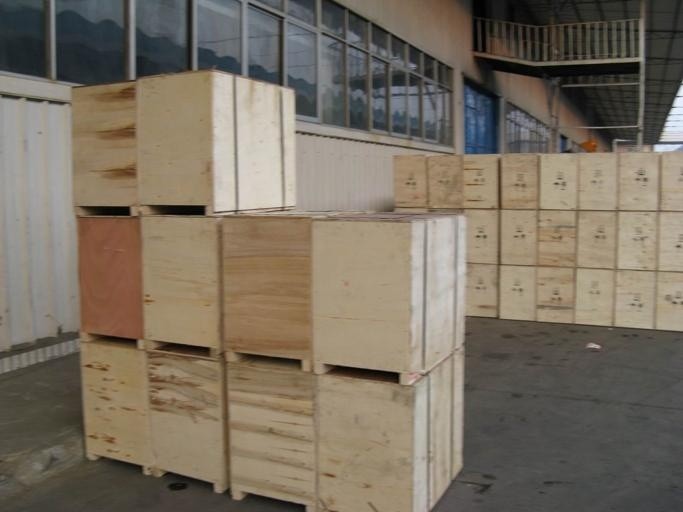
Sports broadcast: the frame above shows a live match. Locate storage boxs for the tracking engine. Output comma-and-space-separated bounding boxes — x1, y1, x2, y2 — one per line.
394, 152, 679, 333
71, 212, 470, 512
63, 71, 303, 216
